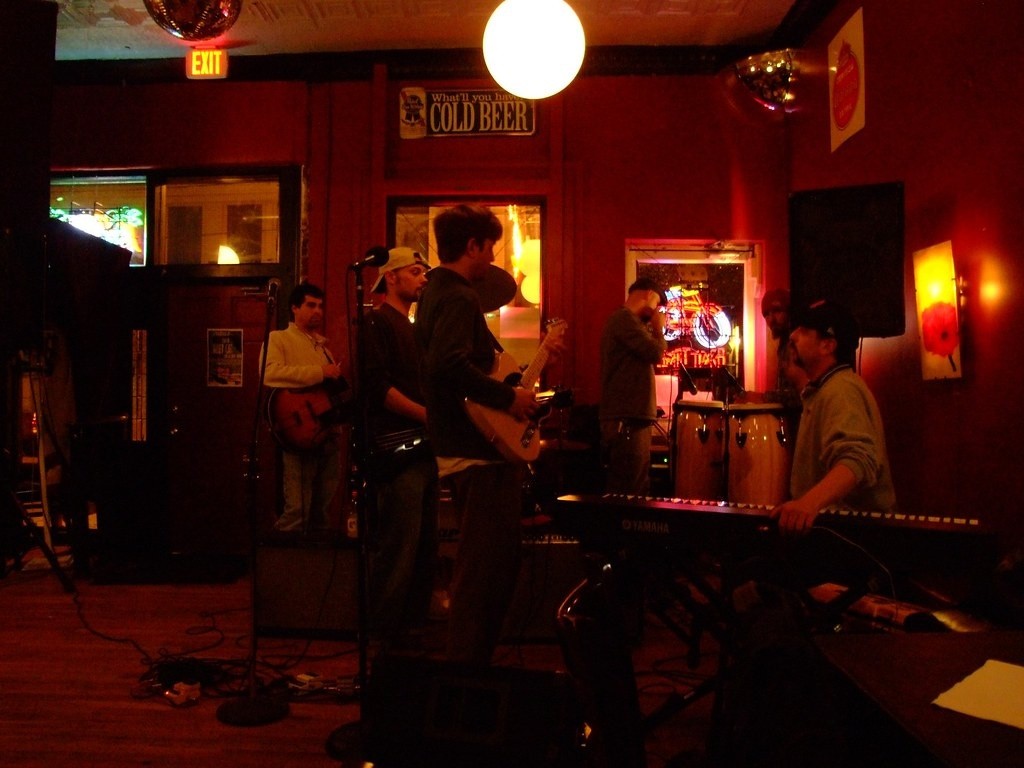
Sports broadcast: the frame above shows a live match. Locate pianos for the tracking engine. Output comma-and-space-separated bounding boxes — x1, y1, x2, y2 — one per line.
366, 385, 571, 461
558, 492, 990, 768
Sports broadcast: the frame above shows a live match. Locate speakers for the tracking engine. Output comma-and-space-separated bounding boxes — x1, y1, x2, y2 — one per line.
360, 653, 606, 768
0, 228, 50, 353
786, 181, 904, 337
250, 546, 362, 638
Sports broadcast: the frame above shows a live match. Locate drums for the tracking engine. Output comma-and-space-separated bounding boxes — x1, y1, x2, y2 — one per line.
726, 400, 793, 503
671, 402, 728, 496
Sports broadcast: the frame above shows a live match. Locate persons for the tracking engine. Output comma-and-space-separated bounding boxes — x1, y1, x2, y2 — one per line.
352, 247, 471, 652
258, 283, 353, 531
760, 285, 897, 538
600, 277, 667, 495
412, 205, 544, 660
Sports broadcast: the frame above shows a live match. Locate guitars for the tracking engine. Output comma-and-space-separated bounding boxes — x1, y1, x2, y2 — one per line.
265, 376, 368, 458
463, 317, 566, 466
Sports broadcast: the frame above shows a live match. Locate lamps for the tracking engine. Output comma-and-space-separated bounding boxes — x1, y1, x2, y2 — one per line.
482, 0, 586, 99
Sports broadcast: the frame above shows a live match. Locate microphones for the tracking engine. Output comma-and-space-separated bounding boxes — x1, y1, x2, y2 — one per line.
721, 367, 744, 394
268, 264, 286, 298
678, 364, 698, 395
348, 246, 389, 269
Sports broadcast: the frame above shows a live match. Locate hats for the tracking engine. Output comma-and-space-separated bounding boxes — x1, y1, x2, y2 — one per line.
799, 300, 859, 351
371, 247, 428, 294
629, 278, 667, 306
